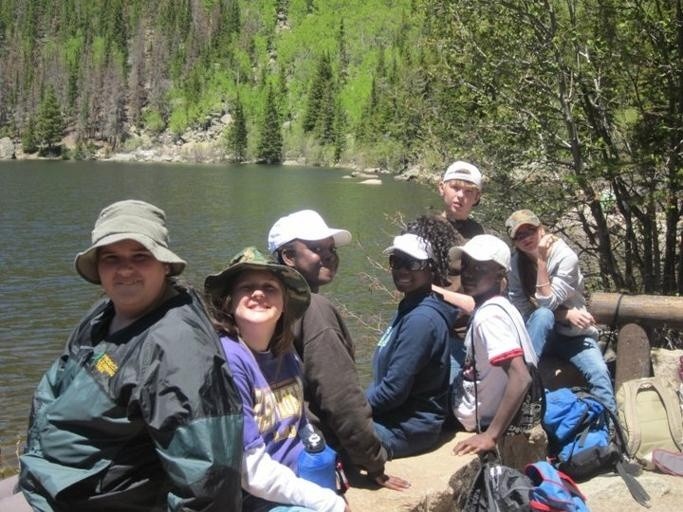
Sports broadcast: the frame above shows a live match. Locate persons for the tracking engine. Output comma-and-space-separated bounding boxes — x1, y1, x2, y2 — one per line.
2, 195, 247, 512
400, 214, 475, 319
263, 208, 414, 495
366, 231, 459, 461
202, 244, 352, 512
505, 210, 623, 442
430, 231, 548, 457
438, 161, 485, 244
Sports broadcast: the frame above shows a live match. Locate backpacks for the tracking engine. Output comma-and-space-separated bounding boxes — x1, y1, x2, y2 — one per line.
612, 375, 682, 474
460, 460, 592, 512
537, 380, 653, 510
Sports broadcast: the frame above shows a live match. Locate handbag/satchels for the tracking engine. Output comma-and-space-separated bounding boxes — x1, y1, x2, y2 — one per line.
542, 374, 626, 478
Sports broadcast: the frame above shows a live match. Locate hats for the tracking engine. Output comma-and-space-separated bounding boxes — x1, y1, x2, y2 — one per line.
204, 247, 311, 317
267, 209, 352, 253
444, 159, 483, 190
504, 209, 542, 239
74, 199, 187, 284
448, 235, 511, 272
383, 233, 435, 260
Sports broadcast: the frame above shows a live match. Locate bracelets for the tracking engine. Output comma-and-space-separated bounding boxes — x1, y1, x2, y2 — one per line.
531, 281, 553, 290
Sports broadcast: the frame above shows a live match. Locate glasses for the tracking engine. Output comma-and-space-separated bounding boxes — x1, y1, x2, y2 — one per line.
514, 225, 540, 240
388, 254, 427, 273
